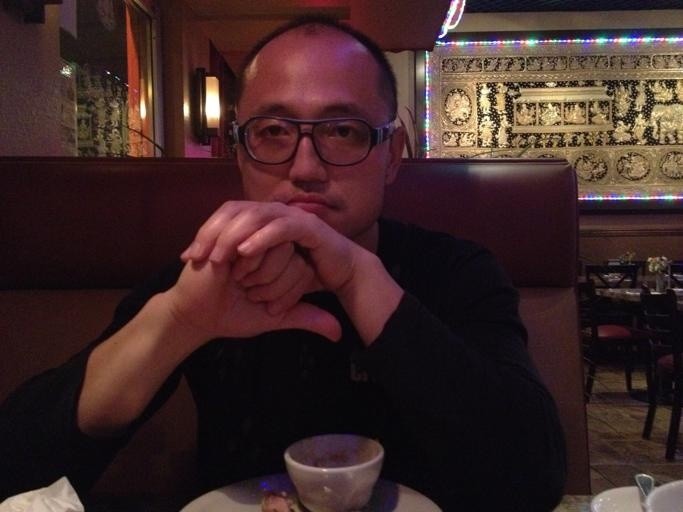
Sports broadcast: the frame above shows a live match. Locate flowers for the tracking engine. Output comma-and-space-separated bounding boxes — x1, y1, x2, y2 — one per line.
647, 256, 671, 274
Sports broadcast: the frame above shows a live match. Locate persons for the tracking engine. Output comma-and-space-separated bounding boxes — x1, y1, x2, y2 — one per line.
0, 12, 572, 510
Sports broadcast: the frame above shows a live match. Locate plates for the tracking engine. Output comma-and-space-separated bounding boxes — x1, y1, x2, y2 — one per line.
179, 474, 683, 512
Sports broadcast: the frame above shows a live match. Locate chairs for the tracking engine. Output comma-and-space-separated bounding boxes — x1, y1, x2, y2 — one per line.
578, 260, 683, 461
1, 156, 592, 495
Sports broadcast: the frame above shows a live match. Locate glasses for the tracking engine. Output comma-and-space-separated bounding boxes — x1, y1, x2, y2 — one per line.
222, 117, 400, 167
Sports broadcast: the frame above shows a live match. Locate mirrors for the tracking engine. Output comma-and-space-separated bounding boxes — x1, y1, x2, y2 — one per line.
76, 0, 129, 157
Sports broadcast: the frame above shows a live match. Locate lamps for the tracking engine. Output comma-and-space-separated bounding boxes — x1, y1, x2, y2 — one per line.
193, 66, 221, 146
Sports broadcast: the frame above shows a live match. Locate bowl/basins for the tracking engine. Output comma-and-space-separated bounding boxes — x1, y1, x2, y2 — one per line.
283, 435, 384, 511
